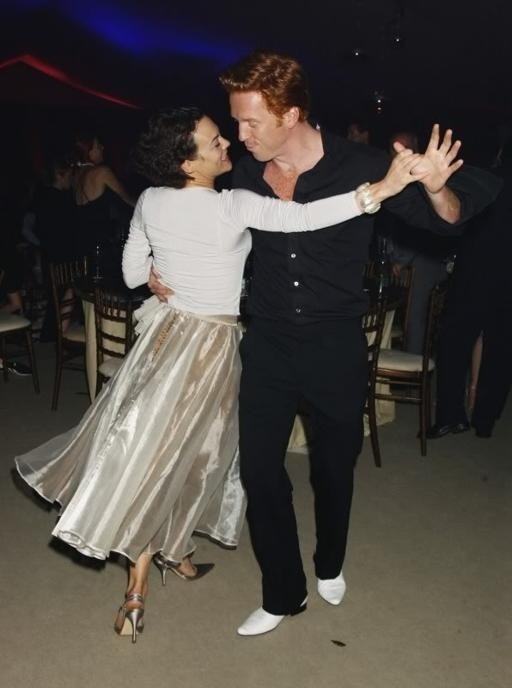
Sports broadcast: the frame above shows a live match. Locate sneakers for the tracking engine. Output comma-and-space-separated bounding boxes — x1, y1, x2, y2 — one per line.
0, 359, 32, 375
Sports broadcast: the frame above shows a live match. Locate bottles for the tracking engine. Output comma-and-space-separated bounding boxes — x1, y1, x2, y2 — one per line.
372, 236, 390, 300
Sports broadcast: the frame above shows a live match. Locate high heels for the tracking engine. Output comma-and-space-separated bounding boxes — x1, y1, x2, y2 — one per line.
154, 555, 215, 584
114, 595, 144, 643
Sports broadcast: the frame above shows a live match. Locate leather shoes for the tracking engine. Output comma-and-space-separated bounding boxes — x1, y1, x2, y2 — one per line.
318, 570, 345, 605
417, 419, 492, 439
237, 595, 308, 635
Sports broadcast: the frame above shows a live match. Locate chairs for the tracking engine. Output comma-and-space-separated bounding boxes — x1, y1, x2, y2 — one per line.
361, 244, 450, 467
3, 245, 134, 410
